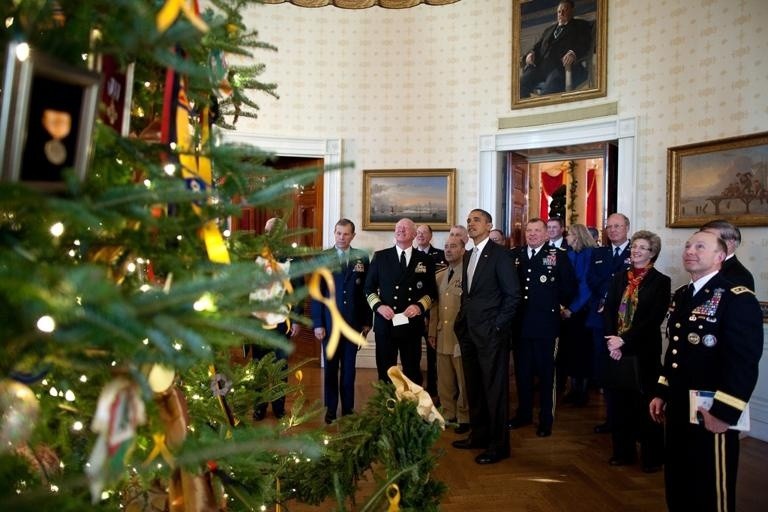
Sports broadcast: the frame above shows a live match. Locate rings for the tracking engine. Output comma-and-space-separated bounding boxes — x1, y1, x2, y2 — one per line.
411, 311, 413, 313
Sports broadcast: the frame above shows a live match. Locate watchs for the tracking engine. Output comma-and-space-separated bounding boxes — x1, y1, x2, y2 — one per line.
619, 336, 625, 345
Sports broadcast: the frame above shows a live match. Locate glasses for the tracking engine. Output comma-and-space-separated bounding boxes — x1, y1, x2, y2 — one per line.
605, 224, 628, 230
628, 244, 652, 251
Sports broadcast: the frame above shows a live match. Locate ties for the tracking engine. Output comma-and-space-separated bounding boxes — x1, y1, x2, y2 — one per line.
551, 242, 556, 247
614, 247, 620, 260
467, 247, 479, 291
530, 249, 535, 259
684, 284, 694, 299
399, 252, 408, 268
447, 269, 454, 283
338, 253, 349, 275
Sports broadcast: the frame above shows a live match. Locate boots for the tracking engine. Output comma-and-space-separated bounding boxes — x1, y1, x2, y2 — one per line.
573, 379, 588, 407
562, 387, 574, 402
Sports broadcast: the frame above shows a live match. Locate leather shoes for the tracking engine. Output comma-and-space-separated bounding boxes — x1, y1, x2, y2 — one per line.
595, 423, 610, 432
476, 447, 511, 463
609, 456, 638, 465
253, 409, 265, 420
537, 426, 553, 436
453, 438, 493, 448
642, 463, 663, 473
508, 417, 532, 427
455, 423, 472, 433
273, 411, 285, 418
324, 411, 336, 423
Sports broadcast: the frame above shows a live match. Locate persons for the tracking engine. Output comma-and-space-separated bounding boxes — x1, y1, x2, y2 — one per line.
511, 219, 574, 438
565, 223, 606, 406
366, 217, 438, 389
602, 231, 673, 469
546, 218, 572, 251
414, 224, 445, 406
243, 217, 304, 422
446, 226, 472, 259
427, 236, 472, 433
521, 1, 598, 102
703, 219, 756, 295
488, 228, 510, 248
648, 230, 764, 512
453, 210, 519, 463
311, 219, 373, 425
594, 212, 630, 434
